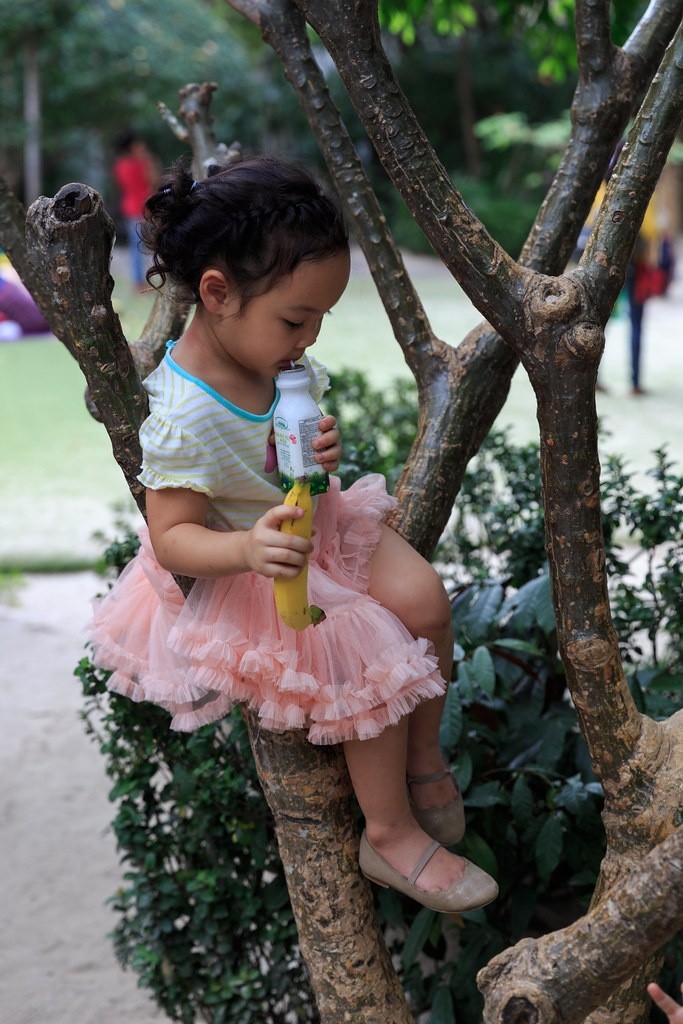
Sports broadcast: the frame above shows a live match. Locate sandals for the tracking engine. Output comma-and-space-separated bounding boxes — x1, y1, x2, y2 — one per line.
359, 766, 499, 916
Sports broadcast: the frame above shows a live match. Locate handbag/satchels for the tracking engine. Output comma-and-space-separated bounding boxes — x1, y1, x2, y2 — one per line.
632, 260, 666, 304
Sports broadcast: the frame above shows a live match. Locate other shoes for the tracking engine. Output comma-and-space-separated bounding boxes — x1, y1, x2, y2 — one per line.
630, 387, 647, 395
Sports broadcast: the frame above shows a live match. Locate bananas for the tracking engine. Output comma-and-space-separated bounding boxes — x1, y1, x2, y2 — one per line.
273, 476, 327, 631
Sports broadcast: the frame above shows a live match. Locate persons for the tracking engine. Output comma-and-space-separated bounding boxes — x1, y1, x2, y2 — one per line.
575, 145, 677, 395
84, 158, 500, 914
114, 140, 160, 293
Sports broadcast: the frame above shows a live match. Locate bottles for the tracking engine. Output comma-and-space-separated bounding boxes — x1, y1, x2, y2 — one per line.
272, 364, 330, 497
